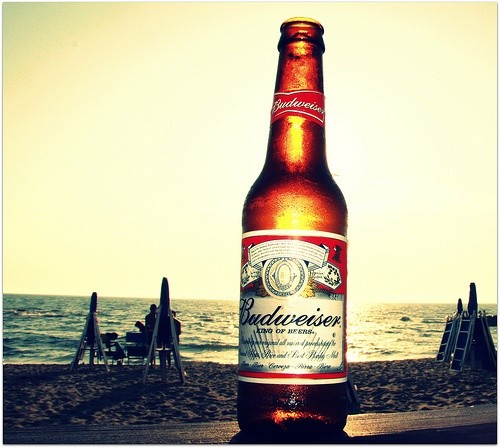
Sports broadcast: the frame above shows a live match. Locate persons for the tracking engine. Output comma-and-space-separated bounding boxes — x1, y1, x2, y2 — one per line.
166, 310, 182, 344
143, 304, 159, 341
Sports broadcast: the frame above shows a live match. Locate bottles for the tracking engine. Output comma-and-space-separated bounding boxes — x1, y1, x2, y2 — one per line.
236, 15, 349, 437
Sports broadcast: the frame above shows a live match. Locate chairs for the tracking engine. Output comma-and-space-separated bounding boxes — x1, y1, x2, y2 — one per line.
154, 335, 178, 371
97, 332, 126, 368
125, 331, 156, 370
